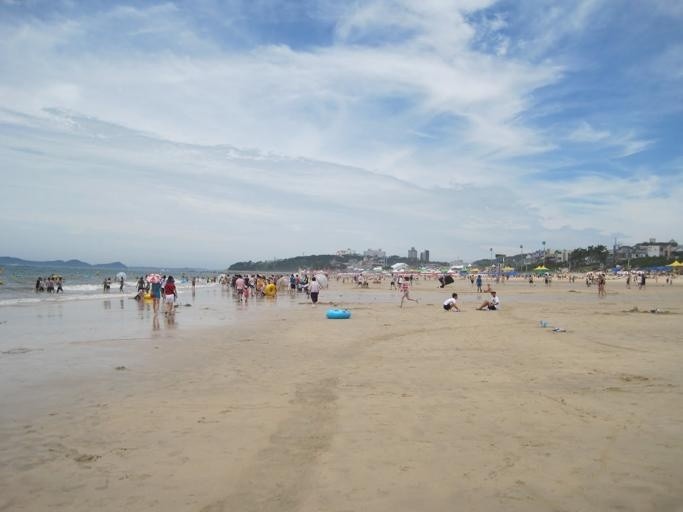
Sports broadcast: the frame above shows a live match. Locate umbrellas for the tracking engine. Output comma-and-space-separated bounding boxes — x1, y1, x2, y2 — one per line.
665, 261, 683, 275
533, 265, 552, 276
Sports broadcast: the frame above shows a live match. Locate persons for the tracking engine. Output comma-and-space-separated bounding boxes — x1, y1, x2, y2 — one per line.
522, 268, 683, 295
442, 293, 461, 312
183, 268, 330, 306
118, 276, 124, 295
34, 274, 63, 293
397, 276, 419, 308
103, 276, 110, 293
334, 267, 518, 289
475, 291, 500, 310
136, 272, 177, 317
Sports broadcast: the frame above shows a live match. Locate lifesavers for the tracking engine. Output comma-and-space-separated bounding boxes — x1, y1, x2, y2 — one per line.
265, 284, 277, 296
143, 293, 152, 300
326, 308, 351, 319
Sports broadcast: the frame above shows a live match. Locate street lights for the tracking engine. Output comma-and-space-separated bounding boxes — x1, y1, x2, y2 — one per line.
542, 241, 546, 269
520, 245, 523, 272
490, 248, 492, 266
